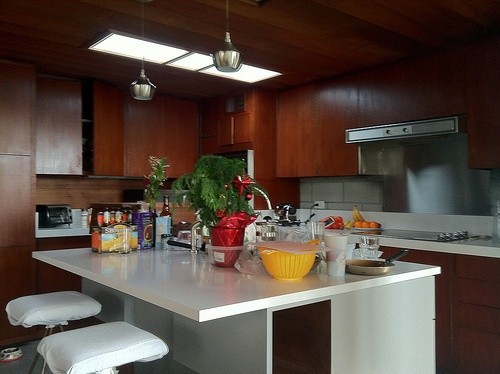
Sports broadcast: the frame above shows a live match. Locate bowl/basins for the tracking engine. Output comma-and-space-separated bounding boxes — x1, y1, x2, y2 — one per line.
255, 241, 321, 281
0, 347, 24, 363
204, 244, 248, 267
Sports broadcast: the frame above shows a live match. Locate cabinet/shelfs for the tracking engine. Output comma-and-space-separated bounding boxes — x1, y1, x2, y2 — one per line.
37, 40, 500, 176
38, 238, 500, 374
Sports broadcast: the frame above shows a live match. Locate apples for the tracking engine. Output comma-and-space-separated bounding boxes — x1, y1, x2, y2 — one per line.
324, 215, 344, 229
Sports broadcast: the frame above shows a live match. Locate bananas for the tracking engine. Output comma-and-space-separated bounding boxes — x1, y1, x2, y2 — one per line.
344, 220, 354, 228
352, 206, 366, 221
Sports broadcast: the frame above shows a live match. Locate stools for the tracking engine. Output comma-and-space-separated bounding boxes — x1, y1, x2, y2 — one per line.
5, 288, 102, 374
36, 320, 169, 374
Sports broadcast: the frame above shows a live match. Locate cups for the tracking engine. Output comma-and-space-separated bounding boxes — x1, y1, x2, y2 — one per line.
357, 236, 379, 259
324, 234, 348, 278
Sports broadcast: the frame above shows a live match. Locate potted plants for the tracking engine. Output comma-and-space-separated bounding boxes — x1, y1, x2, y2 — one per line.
170, 153, 268, 266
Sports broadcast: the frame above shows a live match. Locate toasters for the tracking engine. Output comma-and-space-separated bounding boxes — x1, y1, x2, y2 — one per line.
36, 203, 73, 228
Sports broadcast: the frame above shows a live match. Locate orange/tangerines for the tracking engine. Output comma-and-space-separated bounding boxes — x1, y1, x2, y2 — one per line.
354, 221, 380, 228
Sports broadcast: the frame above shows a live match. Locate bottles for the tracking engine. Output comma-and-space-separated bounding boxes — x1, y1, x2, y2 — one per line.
96, 206, 134, 228
159, 194, 172, 226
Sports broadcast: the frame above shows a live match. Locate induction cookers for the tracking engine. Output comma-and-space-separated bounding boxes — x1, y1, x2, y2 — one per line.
350, 228, 472, 241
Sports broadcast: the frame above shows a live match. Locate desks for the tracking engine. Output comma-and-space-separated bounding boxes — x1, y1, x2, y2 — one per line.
32, 239, 442, 374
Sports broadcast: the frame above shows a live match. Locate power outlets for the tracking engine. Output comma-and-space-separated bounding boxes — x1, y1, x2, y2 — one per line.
314, 201, 325, 209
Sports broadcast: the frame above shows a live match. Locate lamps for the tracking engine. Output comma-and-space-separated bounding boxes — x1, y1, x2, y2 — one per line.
128, 0, 156, 100
212, 0, 243, 72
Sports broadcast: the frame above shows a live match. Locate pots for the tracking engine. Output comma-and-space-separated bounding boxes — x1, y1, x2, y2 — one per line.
345, 246, 410, 275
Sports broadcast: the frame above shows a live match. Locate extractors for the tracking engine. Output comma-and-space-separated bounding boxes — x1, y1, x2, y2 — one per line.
344, 115, 466, 146
